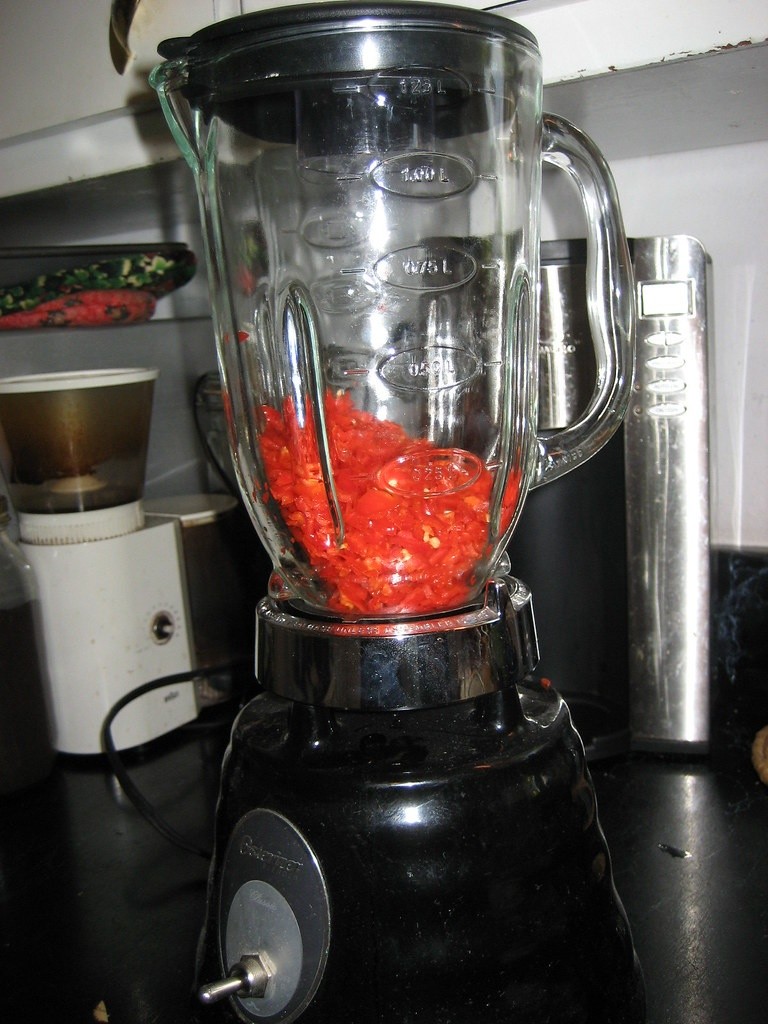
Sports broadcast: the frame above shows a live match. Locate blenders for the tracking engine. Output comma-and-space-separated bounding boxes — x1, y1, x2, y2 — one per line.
148, 0, 658, 1024
1, 368, 200, 768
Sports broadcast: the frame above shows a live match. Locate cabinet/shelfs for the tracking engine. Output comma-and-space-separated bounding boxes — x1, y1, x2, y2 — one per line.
0, 0, 768, 202
